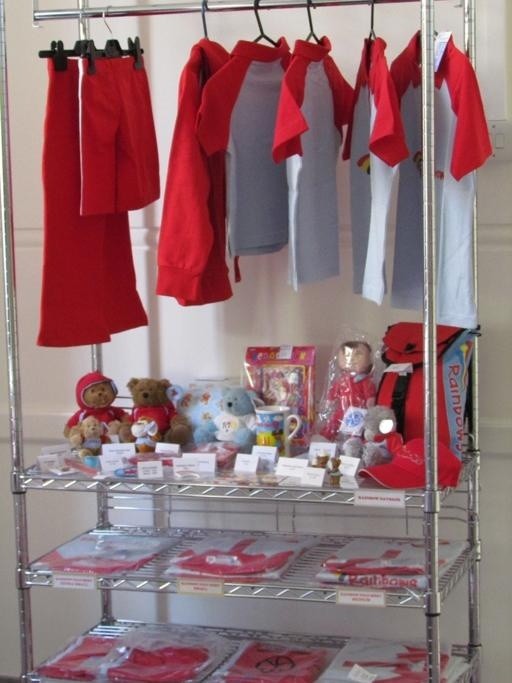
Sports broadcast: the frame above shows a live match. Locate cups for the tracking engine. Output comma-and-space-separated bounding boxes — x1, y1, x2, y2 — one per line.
252, 405, 301, 458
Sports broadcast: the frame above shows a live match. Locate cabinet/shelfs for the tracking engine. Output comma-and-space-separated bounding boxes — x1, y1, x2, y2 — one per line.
0, 1, 482, 682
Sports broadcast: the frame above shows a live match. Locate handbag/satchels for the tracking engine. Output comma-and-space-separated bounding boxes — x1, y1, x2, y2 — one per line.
377, 322, 478, 460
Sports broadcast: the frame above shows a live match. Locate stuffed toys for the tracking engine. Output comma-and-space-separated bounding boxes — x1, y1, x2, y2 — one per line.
341, 403, 396, 464
120, 378, 191, 447
63, 369, 129, 450
186, 386, 264, 453
130, 416, 160, 452
66, 416, 104, 462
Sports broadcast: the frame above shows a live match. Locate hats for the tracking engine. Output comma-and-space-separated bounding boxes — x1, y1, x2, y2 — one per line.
358, 440, 462, 489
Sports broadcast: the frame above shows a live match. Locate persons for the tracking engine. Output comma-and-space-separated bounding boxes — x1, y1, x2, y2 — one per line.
320, 342, 376, 438
